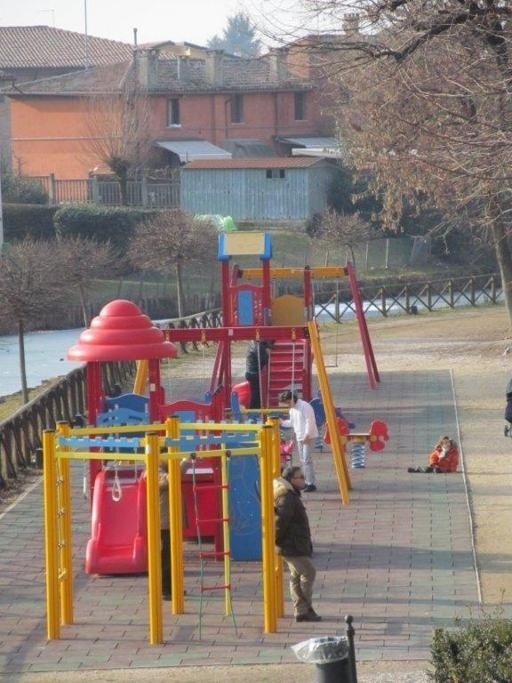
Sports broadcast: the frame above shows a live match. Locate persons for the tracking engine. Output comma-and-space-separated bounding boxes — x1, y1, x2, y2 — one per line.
143, 456, 190, 600
273, 466, 322, 621
408, 436, 459, 474
504, 377, 512, 422
245, 339, 276, 408
278, 390, 319, 492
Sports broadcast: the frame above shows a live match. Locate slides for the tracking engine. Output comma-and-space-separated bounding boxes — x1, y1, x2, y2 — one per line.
86, 469, 148, 576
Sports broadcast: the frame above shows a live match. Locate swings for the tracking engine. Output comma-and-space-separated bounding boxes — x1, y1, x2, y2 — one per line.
313, 278, 341, 368
256, 332, 297, 469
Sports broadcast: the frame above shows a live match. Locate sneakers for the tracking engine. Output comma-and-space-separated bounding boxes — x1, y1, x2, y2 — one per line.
301, 484, 316, 492
296, 608, 322, 622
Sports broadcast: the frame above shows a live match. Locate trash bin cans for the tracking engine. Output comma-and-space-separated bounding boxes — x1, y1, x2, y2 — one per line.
411, 306, 417, 314
309, 636, 351, 682
